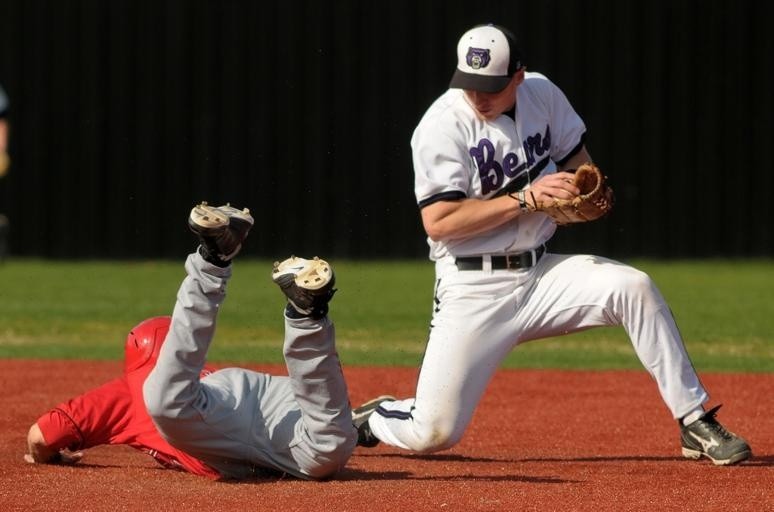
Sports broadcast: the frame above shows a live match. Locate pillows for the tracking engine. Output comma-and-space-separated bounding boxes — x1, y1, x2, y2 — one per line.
124, 315, 171, 386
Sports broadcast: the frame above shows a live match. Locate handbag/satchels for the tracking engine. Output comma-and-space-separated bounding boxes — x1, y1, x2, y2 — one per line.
518, 189, 529, 215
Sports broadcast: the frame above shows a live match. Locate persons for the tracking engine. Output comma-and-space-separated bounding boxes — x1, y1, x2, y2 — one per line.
350, 22, 751, 467
23, 200, 359, 482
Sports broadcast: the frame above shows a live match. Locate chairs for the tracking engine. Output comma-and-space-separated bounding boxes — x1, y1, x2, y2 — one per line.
678, 403, 751, 465
272, 255, 335, 320
187, 202, 254, 262
351, 394, 396, 448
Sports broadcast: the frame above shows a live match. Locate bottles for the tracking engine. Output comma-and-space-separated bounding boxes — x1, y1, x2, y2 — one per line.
449, 22, 524, 93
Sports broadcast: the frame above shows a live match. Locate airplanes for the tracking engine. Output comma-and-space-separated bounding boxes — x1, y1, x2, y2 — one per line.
532, 163, 613, 225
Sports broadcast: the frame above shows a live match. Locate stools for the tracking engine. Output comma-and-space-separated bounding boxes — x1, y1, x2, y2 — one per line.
456, 244, 545, 271
